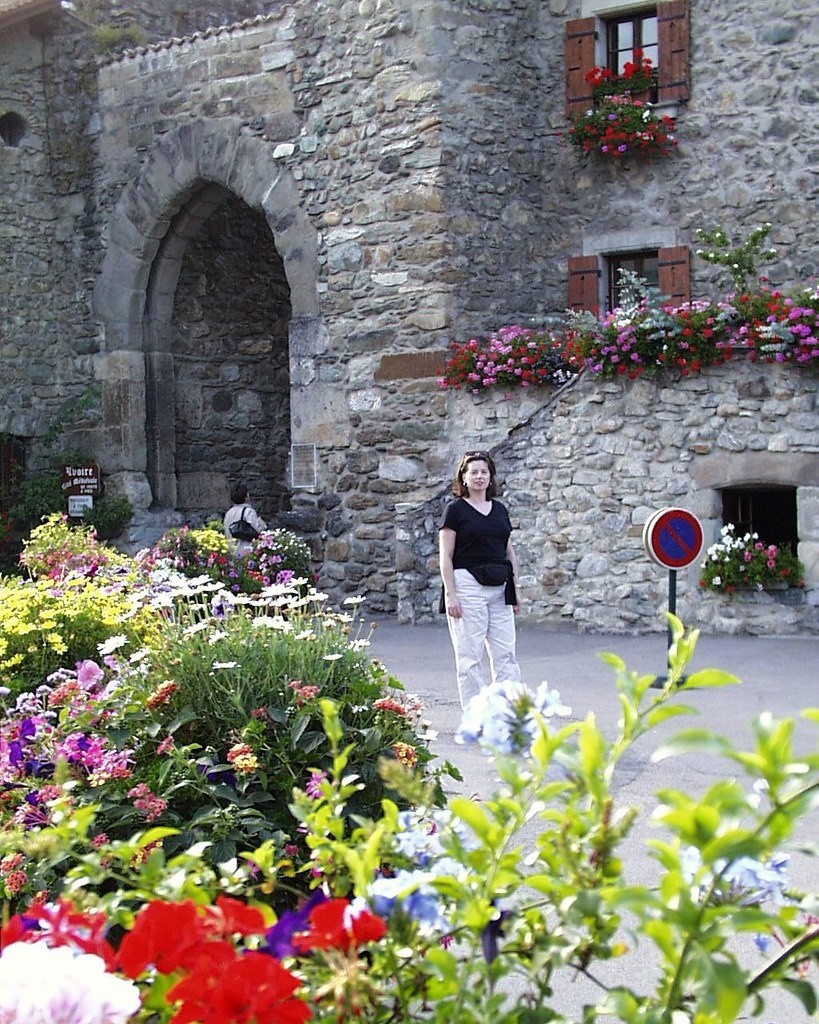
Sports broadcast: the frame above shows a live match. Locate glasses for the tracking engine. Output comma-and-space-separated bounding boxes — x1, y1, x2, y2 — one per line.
468, 450, 490, 457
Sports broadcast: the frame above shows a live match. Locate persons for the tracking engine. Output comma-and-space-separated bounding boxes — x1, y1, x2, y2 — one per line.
223, 485, 266, 554
439, 451, 523, 709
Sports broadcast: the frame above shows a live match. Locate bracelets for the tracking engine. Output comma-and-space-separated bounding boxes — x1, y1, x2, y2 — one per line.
515, 584, 521, 588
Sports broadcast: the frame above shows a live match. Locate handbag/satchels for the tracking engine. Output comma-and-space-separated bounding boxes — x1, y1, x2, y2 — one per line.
466, 557, 509, 586
229, 506, 259, 542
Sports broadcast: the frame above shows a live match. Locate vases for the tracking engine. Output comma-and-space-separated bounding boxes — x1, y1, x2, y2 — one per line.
633, 89, 658, 104
734, 578, 789, 590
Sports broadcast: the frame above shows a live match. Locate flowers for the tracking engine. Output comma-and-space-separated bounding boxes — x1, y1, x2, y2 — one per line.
561, 89, 679, 166
700, 523, 806, 602
436, 220, 819, 403
584, 48, 658, 102
